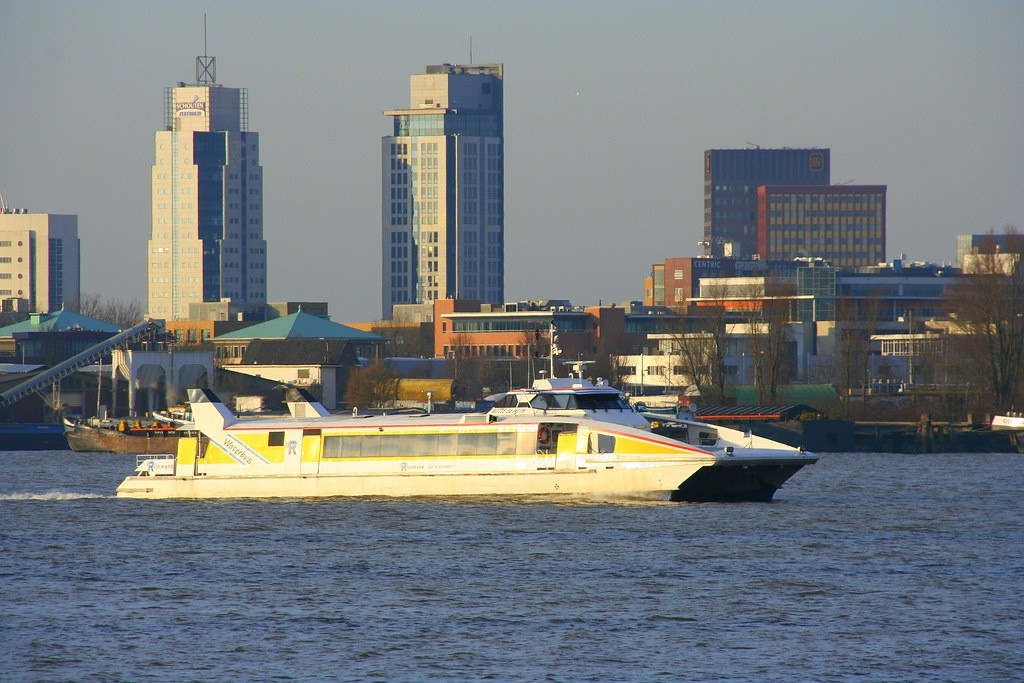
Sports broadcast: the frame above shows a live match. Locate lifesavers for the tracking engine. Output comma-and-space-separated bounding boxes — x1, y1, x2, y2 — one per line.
537, 427, 552, 444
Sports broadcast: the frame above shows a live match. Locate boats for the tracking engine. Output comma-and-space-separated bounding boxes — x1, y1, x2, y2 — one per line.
108, 365, 821, 502
61, 412, 210, 459
